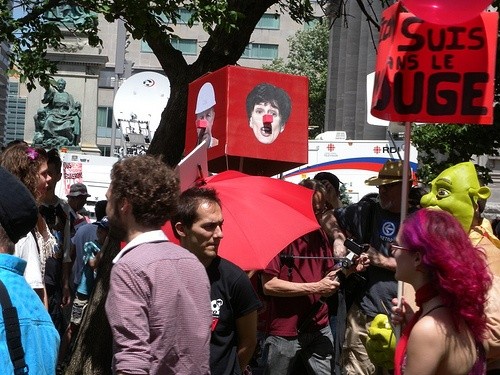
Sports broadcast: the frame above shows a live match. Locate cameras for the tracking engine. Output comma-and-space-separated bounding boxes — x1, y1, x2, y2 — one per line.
342, 239, 365, 269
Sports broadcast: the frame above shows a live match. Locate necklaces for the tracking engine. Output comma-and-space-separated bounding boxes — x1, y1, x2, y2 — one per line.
417, 303, 447, 322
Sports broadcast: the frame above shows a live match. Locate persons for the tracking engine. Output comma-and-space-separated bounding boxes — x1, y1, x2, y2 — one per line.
389, 208, 494, 375
247, 82, 291, 144
104, 153, 212, 375
254, 159, 426, 375
32, 78, 83, 150
419, 161, 500, 375
170, 186, 264, 375
0, 141, 120, 375
194, 82, 219, 149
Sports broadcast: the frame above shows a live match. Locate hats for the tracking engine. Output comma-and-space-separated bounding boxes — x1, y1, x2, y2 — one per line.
0, 165, 38, 243
66, 183, 90, 198
92, 216, 109, 229
313, 172, 341, 191
365, 159, 423, 187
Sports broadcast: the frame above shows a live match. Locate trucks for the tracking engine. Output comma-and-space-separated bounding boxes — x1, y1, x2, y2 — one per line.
263, 138, 418, 202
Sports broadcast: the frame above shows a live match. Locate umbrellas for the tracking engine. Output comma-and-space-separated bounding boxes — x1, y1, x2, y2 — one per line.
120, 169, 322, 273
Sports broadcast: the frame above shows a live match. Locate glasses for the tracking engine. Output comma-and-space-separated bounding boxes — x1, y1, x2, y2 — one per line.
376, 183, 400, 189
389, 242, 411, 253
321, 200, 333, 214
